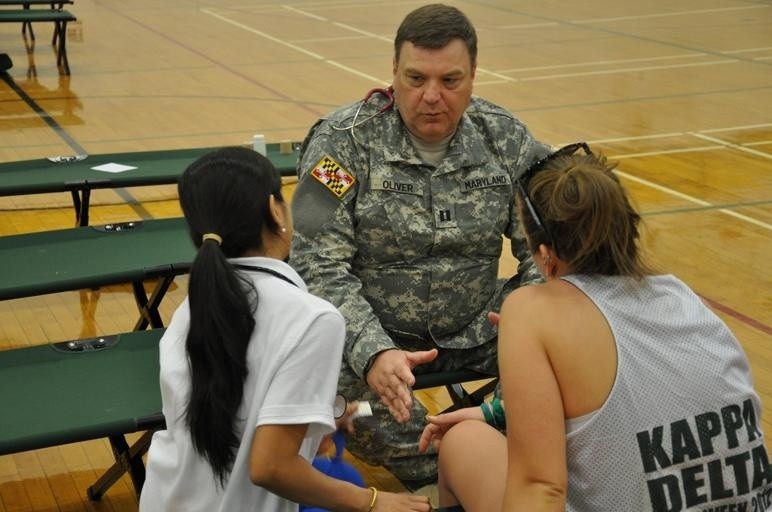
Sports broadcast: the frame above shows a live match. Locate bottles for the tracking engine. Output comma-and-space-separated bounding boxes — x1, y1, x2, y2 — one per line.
253, 134, 266, 157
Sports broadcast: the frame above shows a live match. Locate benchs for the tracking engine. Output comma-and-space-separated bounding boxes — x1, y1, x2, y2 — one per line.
1, 0, 78, 75
0, 141, 502, 501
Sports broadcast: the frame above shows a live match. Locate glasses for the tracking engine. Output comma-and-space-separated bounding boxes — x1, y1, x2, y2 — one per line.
515, 142, 592, 240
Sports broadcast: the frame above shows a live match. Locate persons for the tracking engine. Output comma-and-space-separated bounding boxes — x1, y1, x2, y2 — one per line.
418, 137, 772, 512
287, 0, 562, 497
132, 146, 433, 512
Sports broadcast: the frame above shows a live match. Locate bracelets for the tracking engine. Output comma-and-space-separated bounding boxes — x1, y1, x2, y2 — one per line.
365, 484, 379, 512
480, 401, 499, 432
490, 397, 507, 432
